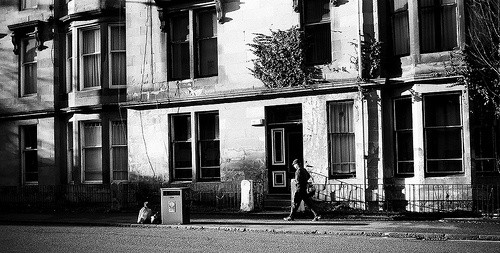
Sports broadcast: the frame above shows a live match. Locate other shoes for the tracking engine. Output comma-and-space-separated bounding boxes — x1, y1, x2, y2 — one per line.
311, 215, 322, 222
284, 216, 293, 220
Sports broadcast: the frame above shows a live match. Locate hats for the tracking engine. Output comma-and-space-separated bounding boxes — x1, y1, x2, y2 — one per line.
292, 159, 300, 165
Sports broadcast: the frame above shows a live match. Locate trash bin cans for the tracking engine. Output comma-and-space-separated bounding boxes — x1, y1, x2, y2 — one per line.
159, 187, 191, 225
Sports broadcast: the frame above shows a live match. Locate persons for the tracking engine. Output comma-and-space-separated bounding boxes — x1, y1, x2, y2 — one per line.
284, 159, 322, 221
